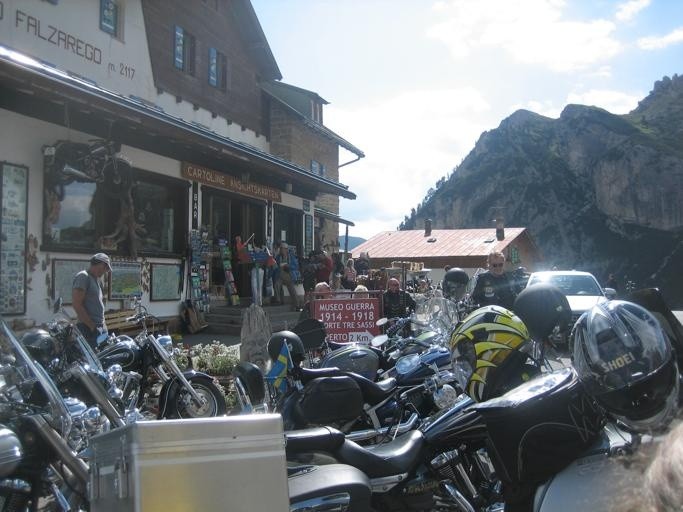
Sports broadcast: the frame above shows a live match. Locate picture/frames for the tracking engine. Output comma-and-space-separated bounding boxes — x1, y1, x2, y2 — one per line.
52, 259, 92, 305
108, 262, 142, 301
0, 162, 29, 315
150, 263, 182, 301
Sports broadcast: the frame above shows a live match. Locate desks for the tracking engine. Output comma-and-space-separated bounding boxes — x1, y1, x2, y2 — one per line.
384, 268, 430, 298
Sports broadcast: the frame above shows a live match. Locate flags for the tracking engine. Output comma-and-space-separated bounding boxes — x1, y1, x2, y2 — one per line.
265, 341, 295, 394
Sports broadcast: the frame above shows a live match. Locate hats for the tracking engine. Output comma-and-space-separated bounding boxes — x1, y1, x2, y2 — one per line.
91, 253, 112, 272
20, 329, 56, 360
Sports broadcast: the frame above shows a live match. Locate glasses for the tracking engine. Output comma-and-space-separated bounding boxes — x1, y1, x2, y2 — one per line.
493, 263, 503, 267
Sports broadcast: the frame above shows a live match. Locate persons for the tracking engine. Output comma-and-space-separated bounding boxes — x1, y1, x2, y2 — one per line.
471, 251, 522, 310
417, 280, 428, 294
72, 253, 112, 348
606, 274, 617, 288
233, 234, 369, 312
383, 278, 418, 318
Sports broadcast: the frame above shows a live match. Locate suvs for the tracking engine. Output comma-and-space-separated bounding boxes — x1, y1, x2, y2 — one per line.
517, 268, 614, 347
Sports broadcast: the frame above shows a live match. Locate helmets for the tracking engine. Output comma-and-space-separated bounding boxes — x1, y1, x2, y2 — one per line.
267, 331, 307, 361
444, 268, 672, 402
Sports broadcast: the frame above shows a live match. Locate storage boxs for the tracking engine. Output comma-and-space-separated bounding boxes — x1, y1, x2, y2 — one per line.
464, 368, 606, 483
87, 413, 290, 512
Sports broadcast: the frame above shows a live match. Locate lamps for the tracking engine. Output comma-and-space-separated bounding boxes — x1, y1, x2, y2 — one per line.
284, 181, 293, 193
240, 169, 250, 184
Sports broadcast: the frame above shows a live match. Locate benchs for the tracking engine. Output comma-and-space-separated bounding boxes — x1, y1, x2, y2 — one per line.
73, 310, 170, 337
